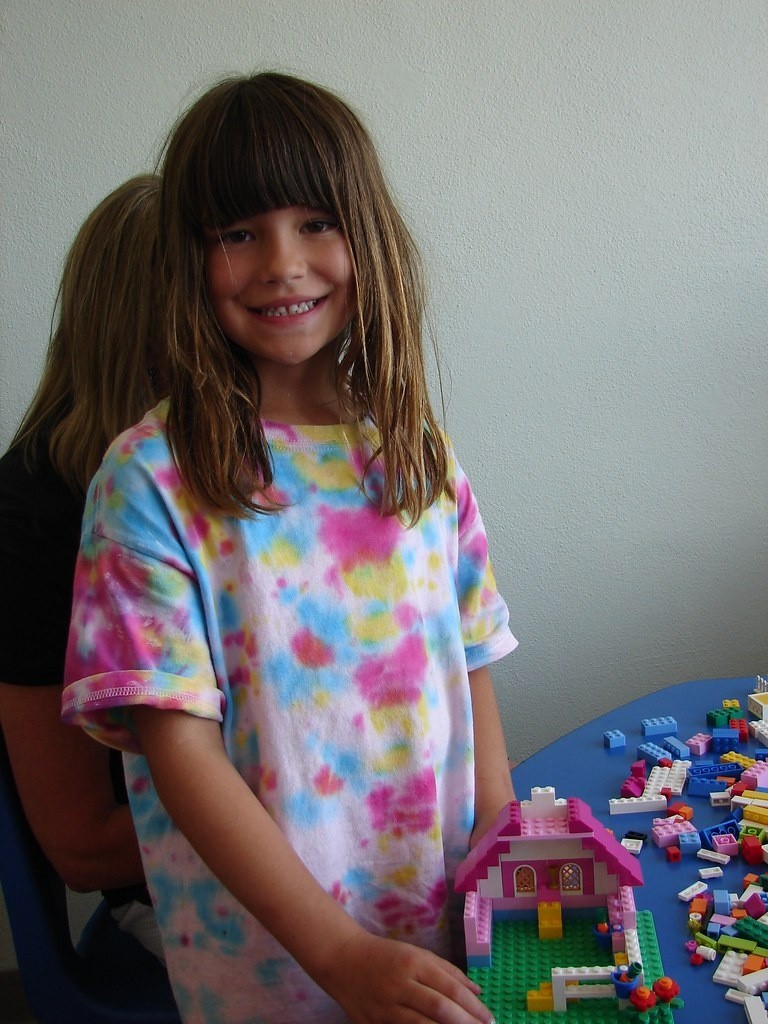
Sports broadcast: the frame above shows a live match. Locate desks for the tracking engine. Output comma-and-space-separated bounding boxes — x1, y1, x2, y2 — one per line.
508, 672, 768, 1024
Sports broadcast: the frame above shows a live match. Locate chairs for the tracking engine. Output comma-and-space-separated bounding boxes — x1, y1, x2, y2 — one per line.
1, 719, 182, 1024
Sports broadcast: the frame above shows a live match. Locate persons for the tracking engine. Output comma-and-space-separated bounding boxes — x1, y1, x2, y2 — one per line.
0, 172, 173, 1024
62, 70, 520, 1024
